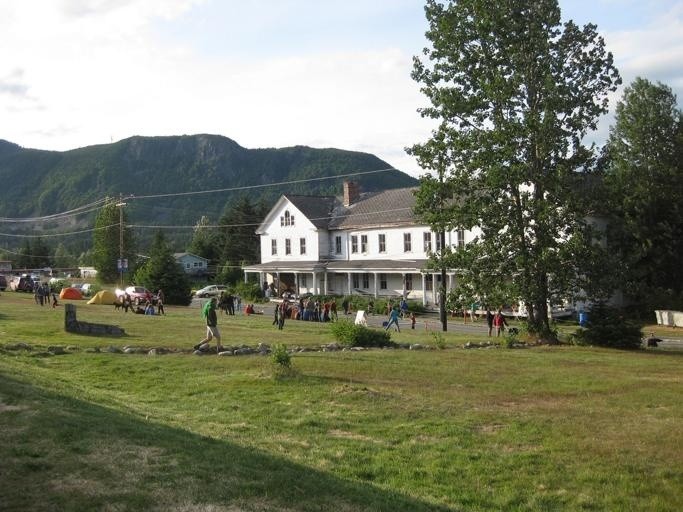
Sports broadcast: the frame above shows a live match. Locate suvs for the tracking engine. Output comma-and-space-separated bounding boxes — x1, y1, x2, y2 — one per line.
22, 273, 41, 288
8, 273, 33, 292
0, 275, 7, 291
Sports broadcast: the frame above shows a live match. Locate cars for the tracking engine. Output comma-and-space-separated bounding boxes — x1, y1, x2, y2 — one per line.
70, 284, 81, 290
49, 277, 65, 286
115, 284, 157, 306
81, 284, 100, 297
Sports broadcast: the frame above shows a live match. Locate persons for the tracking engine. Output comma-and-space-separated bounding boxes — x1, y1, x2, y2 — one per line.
272, 297, 349, 330
384, 299, 415, 333
470, 302, 509, 338
33, 281, 62, 309
217, 291, 264, 316
113, 289, 165, 315
367, 297, 375, 316
193, 298, 224, 352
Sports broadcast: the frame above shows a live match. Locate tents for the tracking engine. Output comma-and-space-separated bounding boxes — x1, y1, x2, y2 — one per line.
59, 287, 83, 300
86, 290, 117, 305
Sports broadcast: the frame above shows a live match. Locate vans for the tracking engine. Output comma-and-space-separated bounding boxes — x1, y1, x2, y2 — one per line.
196, 285, 228, 299
